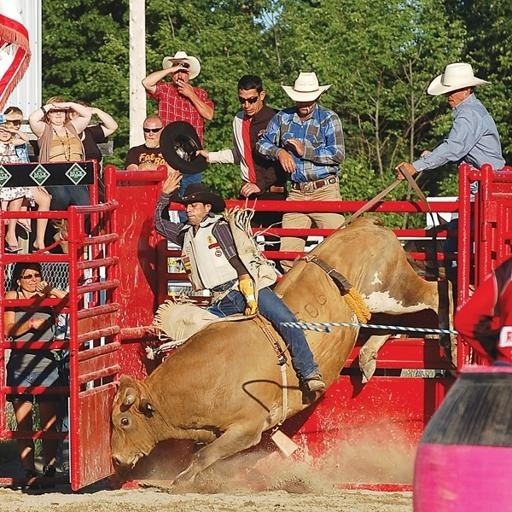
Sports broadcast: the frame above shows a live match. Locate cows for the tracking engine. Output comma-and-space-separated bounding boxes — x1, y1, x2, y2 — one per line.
110, 215, 457, 488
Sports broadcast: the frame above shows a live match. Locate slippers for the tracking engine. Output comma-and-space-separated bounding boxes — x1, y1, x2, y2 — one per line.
32, 250, 48, 253
5, 246, 23, 252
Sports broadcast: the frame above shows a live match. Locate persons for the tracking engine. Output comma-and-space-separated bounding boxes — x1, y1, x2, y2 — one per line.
254, 70, 348, 253
449, 254, 512, 368
3, 263, 68, 495
194, 72, 284, 279
0, 95, 119, 256
124, 116, 169, 174
394, 61, 508, 273
151, 169, 328, 394
141, 49, 215, 201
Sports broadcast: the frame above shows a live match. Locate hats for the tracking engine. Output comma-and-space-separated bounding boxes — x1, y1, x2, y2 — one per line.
162, 51, 201, 79
282, 71, 333, 102
427, 64, 492, 95
160, 121, 208, 173
170, 183, 227, 213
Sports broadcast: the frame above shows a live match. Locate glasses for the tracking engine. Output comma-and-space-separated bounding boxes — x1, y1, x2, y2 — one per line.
239, 96, 258, 104
8, 120, 21, 126
21, 273, 41, 279
143, 128, 162, 132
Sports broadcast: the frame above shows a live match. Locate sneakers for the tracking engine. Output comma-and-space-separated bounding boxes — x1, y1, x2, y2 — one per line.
307, 374, 325, 391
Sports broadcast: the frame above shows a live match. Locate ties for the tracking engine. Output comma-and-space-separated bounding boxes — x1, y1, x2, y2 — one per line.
242, 115, 257, 183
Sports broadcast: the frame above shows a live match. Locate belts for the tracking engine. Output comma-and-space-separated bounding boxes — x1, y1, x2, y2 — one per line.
291, 178, 336, 190
267, 186, 284, 193
